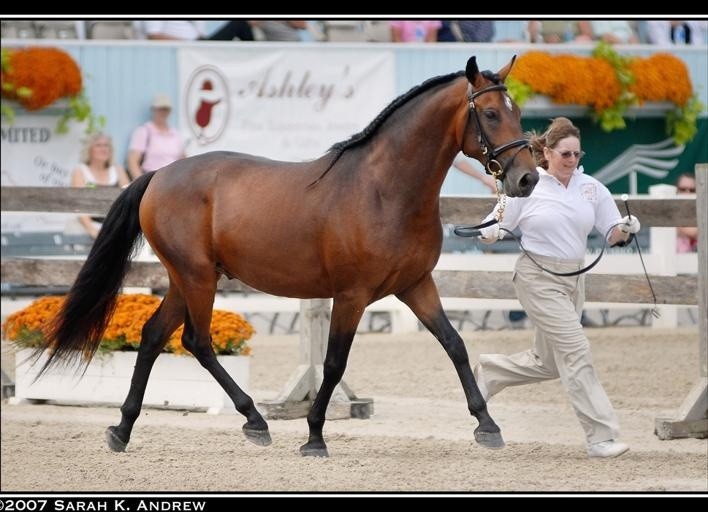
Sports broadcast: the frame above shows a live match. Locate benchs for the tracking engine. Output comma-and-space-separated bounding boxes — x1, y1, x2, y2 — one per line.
1, 227, 98, 263
441, 218, 658, 262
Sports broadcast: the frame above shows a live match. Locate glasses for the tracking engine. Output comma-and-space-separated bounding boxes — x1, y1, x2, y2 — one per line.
551, 148, 584, 159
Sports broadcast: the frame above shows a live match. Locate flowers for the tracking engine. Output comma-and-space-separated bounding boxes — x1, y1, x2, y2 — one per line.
494, 43, 701, 150
0, 48, 102, 136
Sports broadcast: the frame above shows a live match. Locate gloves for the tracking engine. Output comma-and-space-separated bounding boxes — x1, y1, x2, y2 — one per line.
478, 219, 504, 241
620, 215, 640, 234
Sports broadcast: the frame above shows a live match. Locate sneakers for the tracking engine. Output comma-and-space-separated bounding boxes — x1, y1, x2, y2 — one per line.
586, 441, 629, 458
474, 364, 494, 404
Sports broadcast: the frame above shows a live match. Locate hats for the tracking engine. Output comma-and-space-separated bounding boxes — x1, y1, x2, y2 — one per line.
152, 93, 171, 110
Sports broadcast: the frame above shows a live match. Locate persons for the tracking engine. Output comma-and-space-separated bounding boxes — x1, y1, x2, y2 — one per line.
470, 115, 641, 460
4, 22, 705, 45
71, 132, 131, 240
126, 95, 190, 181
674, 176, 698, 253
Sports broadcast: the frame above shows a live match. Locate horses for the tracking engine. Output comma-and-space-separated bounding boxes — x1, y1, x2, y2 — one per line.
2, 54, 540, 458
